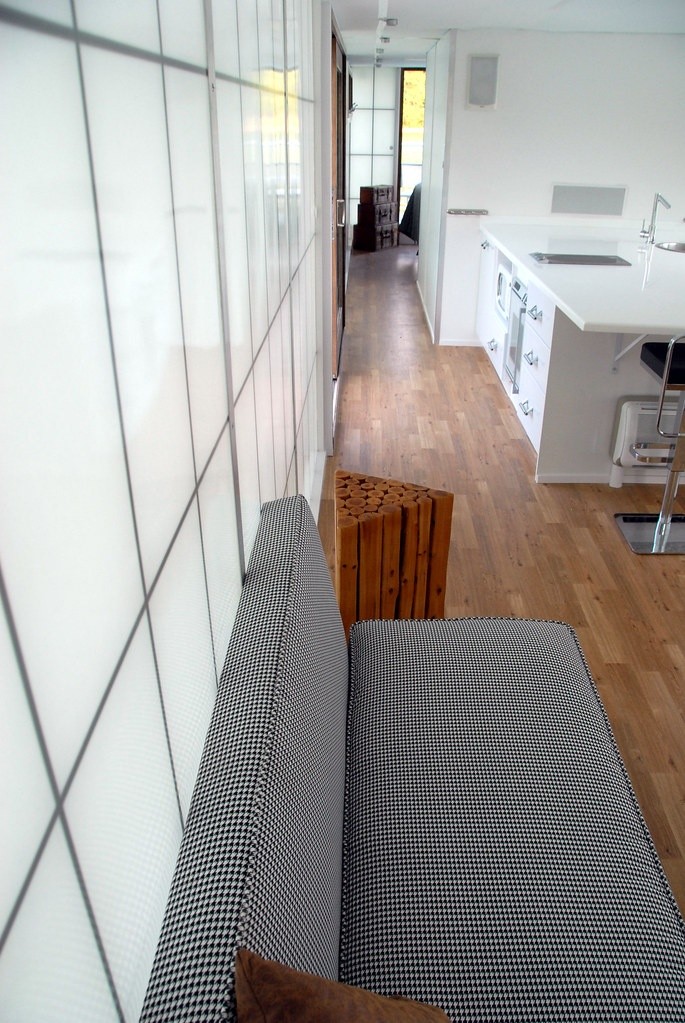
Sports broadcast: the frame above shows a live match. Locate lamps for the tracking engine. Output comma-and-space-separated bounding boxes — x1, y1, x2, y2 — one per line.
375, 18, 398, 66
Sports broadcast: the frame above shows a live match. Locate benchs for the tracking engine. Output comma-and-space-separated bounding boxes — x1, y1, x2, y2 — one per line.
139, 496, 685, 1023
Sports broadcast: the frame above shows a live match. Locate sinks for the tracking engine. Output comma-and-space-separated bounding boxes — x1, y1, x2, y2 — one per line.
654, 242, 685, 253
530, 252, 633, 266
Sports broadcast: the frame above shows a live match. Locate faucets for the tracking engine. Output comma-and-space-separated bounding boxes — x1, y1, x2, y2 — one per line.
640, 192, 671, 244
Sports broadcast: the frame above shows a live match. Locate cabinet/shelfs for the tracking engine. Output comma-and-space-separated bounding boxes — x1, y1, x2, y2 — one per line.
475, 238, 685, 484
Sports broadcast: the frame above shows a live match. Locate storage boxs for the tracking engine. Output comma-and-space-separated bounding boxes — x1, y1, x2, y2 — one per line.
352, 184, 399, 251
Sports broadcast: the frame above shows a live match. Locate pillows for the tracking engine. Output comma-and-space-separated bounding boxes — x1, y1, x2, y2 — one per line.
232, 946, 447, 1023
